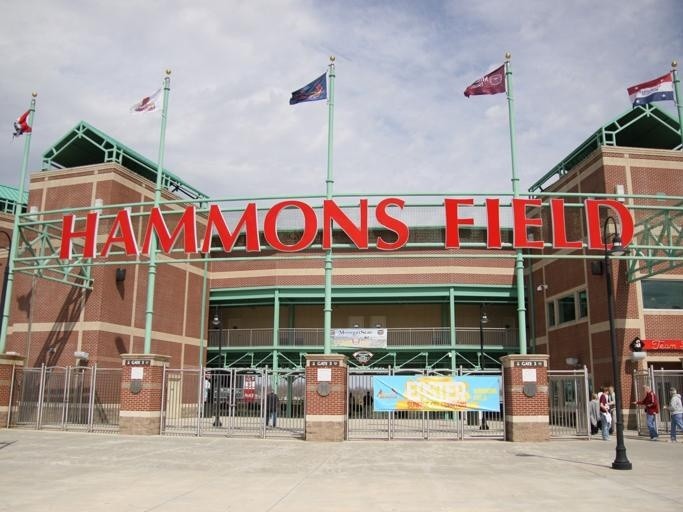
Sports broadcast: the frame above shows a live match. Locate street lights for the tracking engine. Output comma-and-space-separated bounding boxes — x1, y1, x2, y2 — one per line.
212, 302, 223, 428
477, 299, 492, 431
596, 213, 634, 471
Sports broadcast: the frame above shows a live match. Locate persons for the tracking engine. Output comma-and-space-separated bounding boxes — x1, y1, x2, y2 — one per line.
589, 385, 616, 440
663, 386, 683, 441
347, 392, 355, 418
362, 391, 373, 412
630, 385, 658, 441
261, 389, 280, 428
203, 376, 211, 402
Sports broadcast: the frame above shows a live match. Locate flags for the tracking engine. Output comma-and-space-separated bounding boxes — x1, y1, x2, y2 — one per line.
463, 64, 506, 97
626, 71, 675, 108
130, 81, 162, 114
288, 72, 326, 106
10, 108, 35, 138
